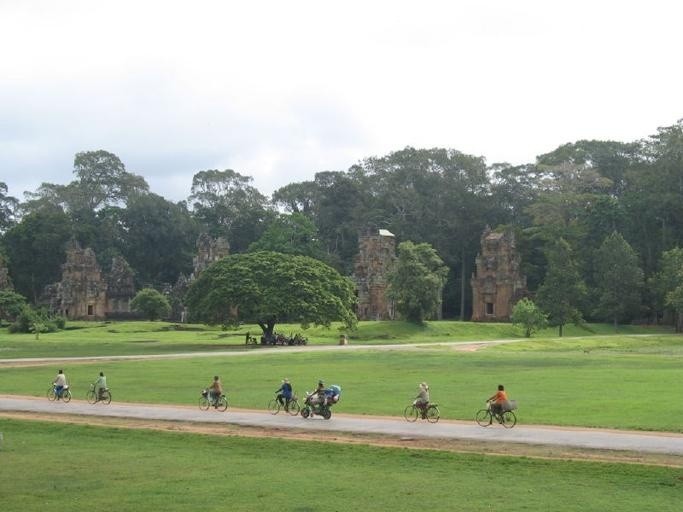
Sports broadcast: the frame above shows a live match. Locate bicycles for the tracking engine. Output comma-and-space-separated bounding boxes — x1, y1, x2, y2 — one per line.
403, 396, 441, 423
475, 398, 518, 429
85, 382, 114, 406
266, 389, 302, 417
198, 385, 229, 412
46, 380, 74, 403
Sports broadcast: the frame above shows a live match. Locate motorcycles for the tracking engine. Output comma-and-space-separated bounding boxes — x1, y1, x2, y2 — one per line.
299, 391, 335, 418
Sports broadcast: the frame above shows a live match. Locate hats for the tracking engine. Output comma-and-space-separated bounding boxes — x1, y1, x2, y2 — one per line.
282, 379, 289, 384
419, 383, 428, 389
318, 379, 324, 385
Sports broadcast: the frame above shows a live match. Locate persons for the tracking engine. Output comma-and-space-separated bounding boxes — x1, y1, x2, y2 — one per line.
50, 369, 67, 402
307, 379, 326, 416
205, 375, 223, 410
92, 371, 107, 404
274, 377, 293, 413
485, 385, 509, 424
411, 382, 431, 420
